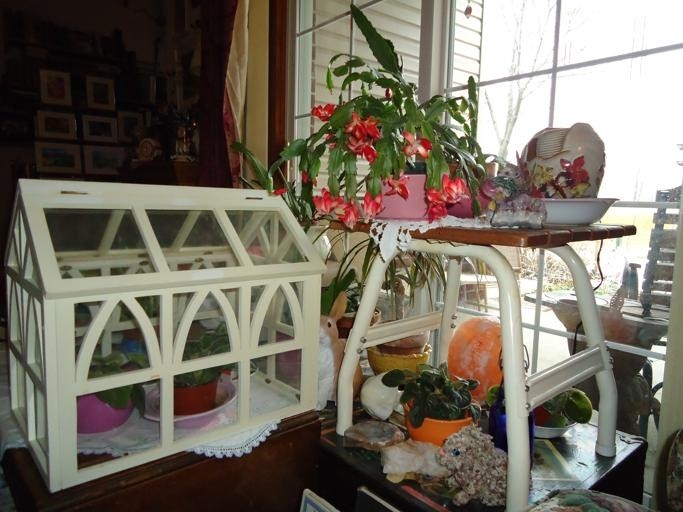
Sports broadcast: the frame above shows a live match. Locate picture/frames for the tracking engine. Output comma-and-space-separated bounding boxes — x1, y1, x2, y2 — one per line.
40, 70, 71, 105
83, 117, 118, 141
37, 113, 77, 139
86, 147, 124, 176
87, 77, 115, 109
34, 143, 82, 175
119, 113, 143, 140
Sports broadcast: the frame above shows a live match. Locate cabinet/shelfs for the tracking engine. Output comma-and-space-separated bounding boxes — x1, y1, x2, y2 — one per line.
0, 408, 318, 512
320, 407, 647, 512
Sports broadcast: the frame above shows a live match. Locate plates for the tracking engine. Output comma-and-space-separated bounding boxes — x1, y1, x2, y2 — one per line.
143, 379, 238, 430
533, 418, 577, 438
532, 197, 619, 227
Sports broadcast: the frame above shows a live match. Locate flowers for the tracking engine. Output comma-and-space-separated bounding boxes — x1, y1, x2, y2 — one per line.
268, 2, 483, 229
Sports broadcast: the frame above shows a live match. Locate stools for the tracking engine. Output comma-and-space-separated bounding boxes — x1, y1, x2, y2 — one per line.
336, 223, 634, 512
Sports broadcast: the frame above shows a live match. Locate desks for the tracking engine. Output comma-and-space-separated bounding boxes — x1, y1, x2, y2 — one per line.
524, 290, 670, 410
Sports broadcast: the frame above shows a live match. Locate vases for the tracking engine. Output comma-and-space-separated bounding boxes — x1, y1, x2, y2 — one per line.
365, 176, 431, 218
445, 162, 499, 220
366, 347, 432, 386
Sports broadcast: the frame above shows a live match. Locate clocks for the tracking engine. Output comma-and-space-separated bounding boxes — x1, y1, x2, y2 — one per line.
136, 138, 160, 160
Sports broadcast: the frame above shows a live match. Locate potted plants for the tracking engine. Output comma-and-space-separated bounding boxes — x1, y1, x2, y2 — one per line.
311, 212, 488, 354
174, 318, 256, 417
76, 353, 149, 430
486, 379, 592, 439
381, 366, 481, 446
